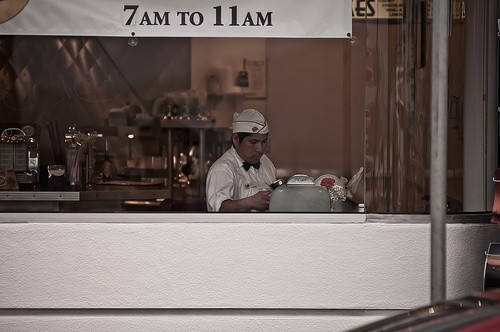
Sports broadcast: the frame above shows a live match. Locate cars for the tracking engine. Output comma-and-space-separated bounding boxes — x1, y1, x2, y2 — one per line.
337, 287, 500, 332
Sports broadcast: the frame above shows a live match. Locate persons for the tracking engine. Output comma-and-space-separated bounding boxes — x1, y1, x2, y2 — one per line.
206, 108, 277, 213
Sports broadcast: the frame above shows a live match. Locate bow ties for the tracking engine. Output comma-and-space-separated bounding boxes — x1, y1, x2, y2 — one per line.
242, 161, 261, 171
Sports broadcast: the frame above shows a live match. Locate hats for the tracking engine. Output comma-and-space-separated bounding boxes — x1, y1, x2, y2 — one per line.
232, 109, 269, 134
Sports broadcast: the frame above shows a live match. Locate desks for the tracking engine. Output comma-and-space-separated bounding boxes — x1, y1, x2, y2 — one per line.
0, 185, 171, 213
